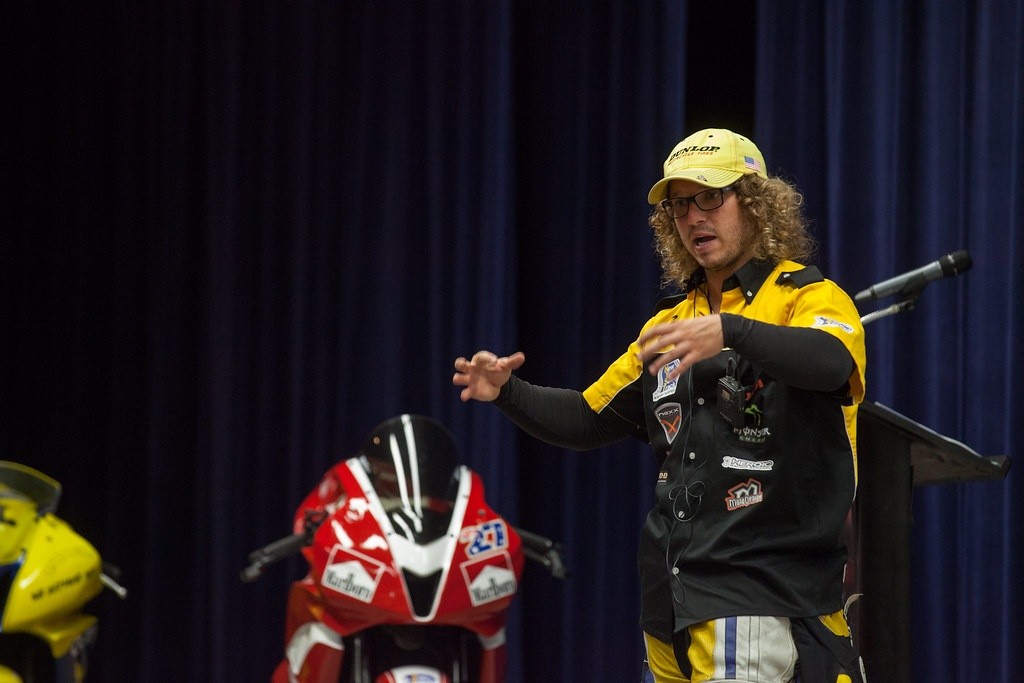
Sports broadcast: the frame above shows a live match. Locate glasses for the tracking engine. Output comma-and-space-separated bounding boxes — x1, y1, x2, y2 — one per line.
662, 187, 737, 218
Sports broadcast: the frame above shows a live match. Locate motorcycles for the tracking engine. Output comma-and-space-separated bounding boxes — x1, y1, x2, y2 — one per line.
232, 430, 555, 683
0, 459, 129, 683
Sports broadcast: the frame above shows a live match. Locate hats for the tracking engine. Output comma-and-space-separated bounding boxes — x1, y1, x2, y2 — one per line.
647, 128, 767, 204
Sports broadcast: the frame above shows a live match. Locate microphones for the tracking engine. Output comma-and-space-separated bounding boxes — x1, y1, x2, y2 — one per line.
855, 248, 974, 305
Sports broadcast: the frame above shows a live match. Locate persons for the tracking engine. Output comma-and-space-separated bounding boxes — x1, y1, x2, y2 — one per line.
450, 126, 868, 682
272, 404, 450, 682
